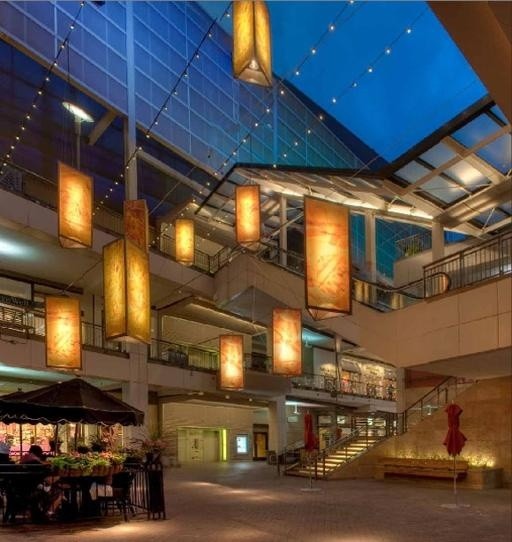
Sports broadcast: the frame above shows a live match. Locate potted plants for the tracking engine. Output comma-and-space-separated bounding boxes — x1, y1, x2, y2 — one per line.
53, 453, 126, 475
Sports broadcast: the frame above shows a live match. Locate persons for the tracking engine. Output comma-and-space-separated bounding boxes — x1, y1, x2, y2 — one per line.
20, 445, 64, 522
89, 466, 114, 501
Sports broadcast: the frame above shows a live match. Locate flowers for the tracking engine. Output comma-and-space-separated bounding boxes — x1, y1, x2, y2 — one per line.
130, 424, 179, 452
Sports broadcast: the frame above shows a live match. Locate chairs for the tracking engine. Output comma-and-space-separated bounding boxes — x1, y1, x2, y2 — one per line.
0, 468, 136, 522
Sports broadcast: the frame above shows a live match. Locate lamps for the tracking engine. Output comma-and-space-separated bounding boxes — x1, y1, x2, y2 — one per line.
193, 5, 230, 59
207, 151, 237, 187
218, 207, 235, 224
152, 223, 173, 244
45, 290, 82, 376
124, 194, 149, 250
175, 209, 196, 272
271, 303, 303, 378
265, 142, 298, 179
180, 191, 202, 215
307, 99, 337, 134
296, 24, 335, 78
103, 226, 151, 349
303, 186, 350, 322
233, 0, 274, 88
243, 88, 285, 144
235, 171, 260, 254
43, 2, 85, 87
218, 327, 245, 390
58, 155, 94, 252
197, 228, 215, 249
135, 60, 192, 154
95, 155, 136, 220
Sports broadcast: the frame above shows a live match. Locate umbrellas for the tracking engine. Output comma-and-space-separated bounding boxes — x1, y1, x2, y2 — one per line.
303, 409, 319, 489
0, 373, 145, 458
441, 399, 467, 505
